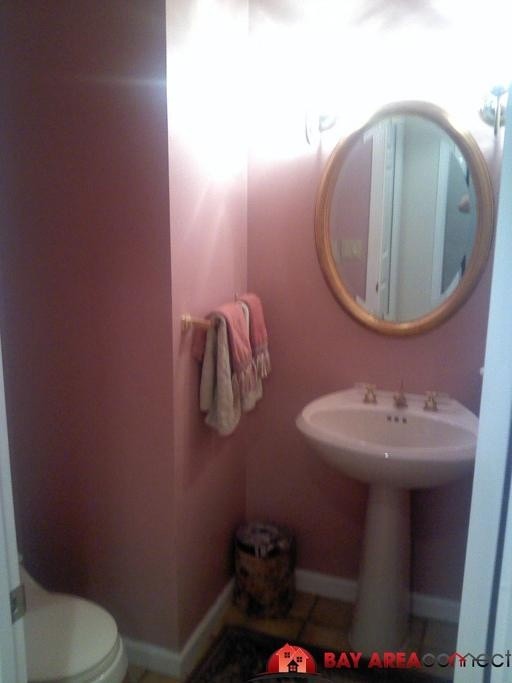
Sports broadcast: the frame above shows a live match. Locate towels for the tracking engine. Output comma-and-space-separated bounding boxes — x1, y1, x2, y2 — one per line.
190, 292, 271, 438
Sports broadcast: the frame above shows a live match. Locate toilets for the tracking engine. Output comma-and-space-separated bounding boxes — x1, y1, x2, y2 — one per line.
17, 561, 129, 683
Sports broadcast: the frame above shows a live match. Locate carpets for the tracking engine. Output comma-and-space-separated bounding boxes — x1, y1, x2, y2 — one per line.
182, 621, 452, 683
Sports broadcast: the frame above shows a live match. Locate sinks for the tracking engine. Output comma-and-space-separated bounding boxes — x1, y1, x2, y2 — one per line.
293, 387, 479, 491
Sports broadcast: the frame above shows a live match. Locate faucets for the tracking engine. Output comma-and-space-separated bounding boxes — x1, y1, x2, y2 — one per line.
390, 381, 407, 409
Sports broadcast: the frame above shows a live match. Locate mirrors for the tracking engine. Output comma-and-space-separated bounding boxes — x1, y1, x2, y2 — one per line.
312, 99, 496, 338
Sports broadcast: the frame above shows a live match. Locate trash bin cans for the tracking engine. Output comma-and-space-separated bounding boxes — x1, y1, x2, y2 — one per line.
234, 521, 296, 619
